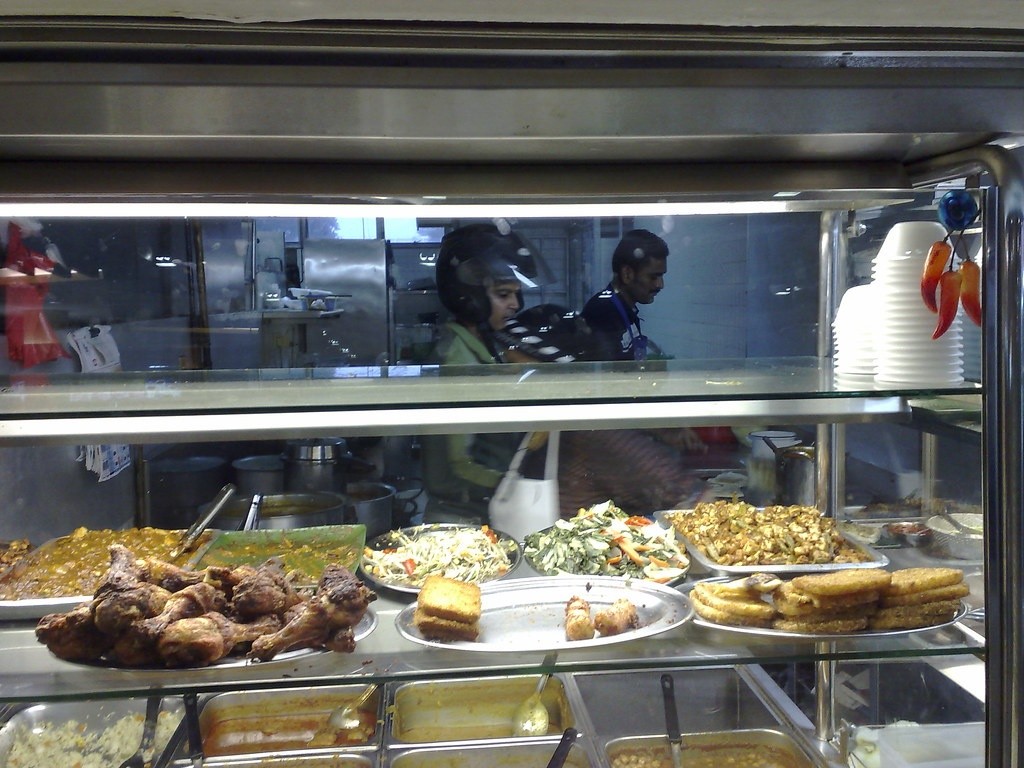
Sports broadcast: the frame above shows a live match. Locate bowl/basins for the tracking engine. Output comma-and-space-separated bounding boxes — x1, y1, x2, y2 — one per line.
883, 523, 929, 548
832, 220, 958, 385
925, 513, 986, 560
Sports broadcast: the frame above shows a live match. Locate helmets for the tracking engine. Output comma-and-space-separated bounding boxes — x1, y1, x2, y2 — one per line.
493, 304, 592, 362
436, 224, 538, 325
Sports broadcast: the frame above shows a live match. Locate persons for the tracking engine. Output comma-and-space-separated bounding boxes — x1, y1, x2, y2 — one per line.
419, 223, 706, 523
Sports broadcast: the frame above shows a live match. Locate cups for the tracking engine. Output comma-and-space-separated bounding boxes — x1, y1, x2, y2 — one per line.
749, 429, 795, 459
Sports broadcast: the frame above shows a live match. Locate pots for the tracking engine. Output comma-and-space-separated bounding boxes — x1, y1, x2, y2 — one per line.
281, 436, 355, 493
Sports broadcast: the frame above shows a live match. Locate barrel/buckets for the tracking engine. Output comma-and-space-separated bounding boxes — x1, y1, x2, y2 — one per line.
233, 455, 284, 494
347, 482, 396, 539
148, 457, 229, 529
196, 492, 344, 529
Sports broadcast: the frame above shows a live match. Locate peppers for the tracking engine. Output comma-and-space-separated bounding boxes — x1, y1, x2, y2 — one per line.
959, 237, 981, 326
921, 241, 951, 312
932, 271, 961, 340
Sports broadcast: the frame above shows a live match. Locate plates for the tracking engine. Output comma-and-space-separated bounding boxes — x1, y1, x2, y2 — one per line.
673, 577, 966, 639
394, 573, 693, 653
524, 525, 692, 586
197, 523, 367, 591
101, 597, 378, 672
361, 524, 523, 595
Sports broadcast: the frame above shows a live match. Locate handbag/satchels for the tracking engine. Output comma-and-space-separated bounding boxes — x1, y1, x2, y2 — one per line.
488, 430, 561, 542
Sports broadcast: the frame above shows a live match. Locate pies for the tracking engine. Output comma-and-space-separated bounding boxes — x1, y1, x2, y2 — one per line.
416, 606, 479, 639
420, 576, 481, 622
690, 564, 970, 633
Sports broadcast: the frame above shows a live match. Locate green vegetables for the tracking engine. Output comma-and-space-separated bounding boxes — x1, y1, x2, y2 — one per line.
523, 505, 673, 578
